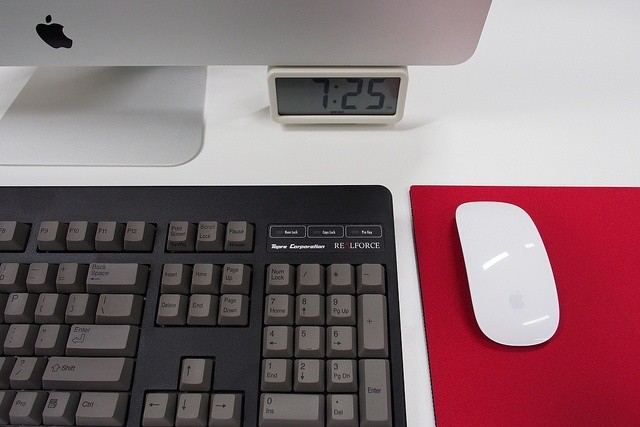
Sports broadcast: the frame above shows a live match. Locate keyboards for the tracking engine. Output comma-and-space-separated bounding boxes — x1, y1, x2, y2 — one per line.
1, 186, 407, 425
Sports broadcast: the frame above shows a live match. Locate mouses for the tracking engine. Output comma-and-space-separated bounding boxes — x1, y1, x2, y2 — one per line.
456, 200, 559, 346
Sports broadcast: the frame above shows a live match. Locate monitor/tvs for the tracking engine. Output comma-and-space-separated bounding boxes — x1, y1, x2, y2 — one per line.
1, 1, 491, 166
